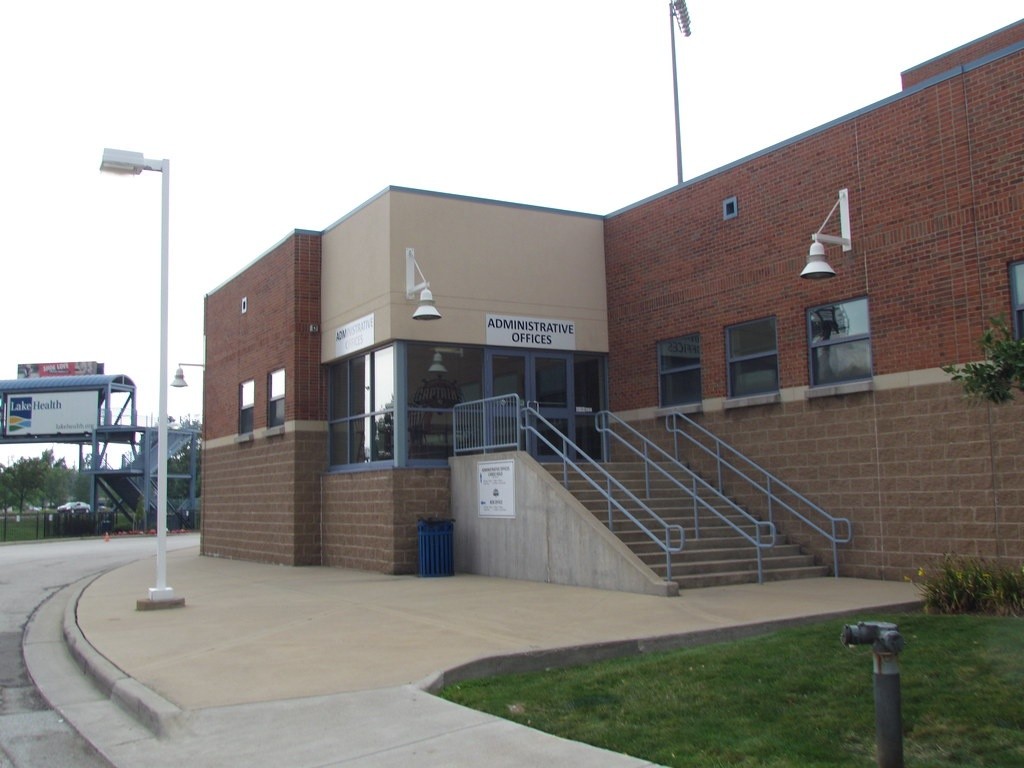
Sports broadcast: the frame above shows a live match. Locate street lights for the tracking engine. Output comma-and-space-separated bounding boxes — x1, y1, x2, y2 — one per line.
96, 146, 183, 611
668, 0, 693, 189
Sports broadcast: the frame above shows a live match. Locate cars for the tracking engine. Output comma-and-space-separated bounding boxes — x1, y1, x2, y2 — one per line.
57, 501, 91, 515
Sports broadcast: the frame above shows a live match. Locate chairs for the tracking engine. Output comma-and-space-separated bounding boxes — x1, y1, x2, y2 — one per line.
354, 402, 429, 464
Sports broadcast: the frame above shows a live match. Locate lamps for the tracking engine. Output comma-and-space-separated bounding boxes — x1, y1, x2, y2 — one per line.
429, 347, 464, 372
799, 189, 851, 281
404, 248, 442, 321
171, 364, 205, 387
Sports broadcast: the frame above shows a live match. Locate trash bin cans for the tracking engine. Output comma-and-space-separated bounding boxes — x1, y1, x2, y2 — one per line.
417, 518, 455, 577
